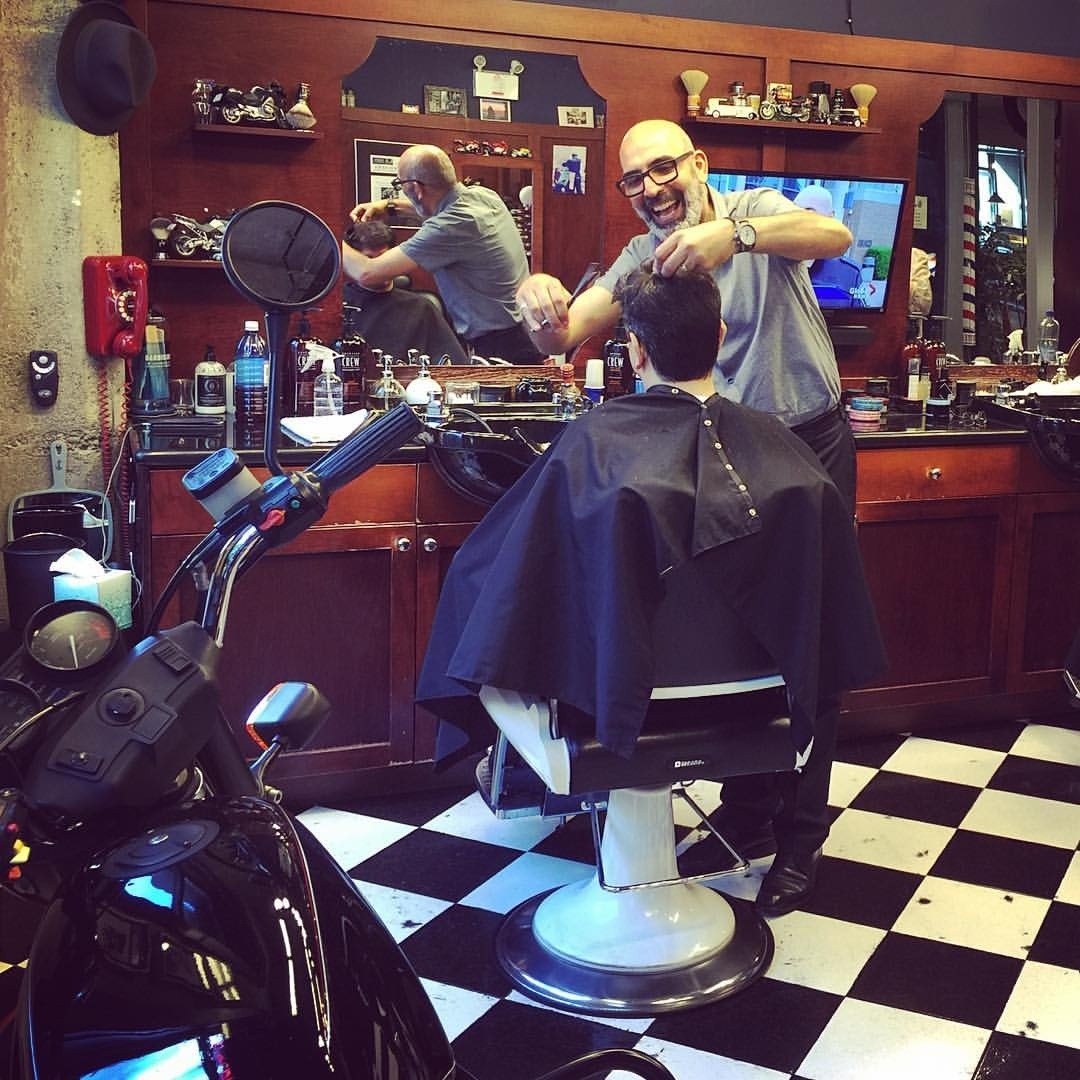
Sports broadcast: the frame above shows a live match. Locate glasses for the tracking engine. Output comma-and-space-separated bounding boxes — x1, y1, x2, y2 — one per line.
392, 178, 424, 191
617, 152, 697, 197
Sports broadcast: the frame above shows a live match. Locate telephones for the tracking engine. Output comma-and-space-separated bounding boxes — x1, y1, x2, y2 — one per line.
83, 255, 149, 359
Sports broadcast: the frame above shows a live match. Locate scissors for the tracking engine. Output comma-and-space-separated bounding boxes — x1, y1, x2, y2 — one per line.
530, 261, 600, 333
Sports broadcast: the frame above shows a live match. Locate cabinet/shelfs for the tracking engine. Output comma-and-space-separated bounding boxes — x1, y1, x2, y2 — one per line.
132, 435, 506, 798
147, 119, 321, 273
813, 423, 1079, 729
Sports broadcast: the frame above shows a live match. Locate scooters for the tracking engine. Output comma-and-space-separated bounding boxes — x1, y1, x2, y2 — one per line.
0, 198, 672, 1080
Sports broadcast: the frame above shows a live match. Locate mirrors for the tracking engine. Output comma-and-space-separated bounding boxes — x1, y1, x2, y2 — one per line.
339, 121, 543, 368
907, 89, 1080, 367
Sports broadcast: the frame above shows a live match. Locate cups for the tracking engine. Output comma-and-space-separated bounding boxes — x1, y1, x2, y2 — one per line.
443, 379, 553, 404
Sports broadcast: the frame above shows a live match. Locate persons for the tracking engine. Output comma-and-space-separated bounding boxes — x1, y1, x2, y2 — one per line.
343, 221, 471, 367
516, 118, 857, 917
791, 185, 870, 309
556, 154, 582, 195
475, 258, 825, 809
342, 144, 545, 366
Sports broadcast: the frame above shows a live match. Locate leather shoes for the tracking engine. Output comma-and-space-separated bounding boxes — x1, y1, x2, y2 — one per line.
756, 845, 824, 913
677, 813, 778, 878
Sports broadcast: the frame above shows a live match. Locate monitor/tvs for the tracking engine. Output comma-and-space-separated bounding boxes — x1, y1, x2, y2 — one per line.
699, 170, 912, 313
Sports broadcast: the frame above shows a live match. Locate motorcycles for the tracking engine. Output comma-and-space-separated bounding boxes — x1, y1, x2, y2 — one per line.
163, 208, 241, 264
759, 85, 811, 124
209, 79, 287, 129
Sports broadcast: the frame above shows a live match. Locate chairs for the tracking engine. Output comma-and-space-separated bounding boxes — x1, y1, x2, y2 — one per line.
490, 476, 831, 1017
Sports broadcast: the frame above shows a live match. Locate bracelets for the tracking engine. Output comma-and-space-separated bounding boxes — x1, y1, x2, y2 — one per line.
386, 198, 397, 217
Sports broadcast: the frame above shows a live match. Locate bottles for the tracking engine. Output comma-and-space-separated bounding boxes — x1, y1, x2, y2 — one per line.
235, 321, 272, 423
1041, 312, 1059, 363
553, 364, 583, 420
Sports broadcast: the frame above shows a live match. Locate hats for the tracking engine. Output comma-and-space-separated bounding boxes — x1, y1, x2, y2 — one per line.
56, 1, 156, 136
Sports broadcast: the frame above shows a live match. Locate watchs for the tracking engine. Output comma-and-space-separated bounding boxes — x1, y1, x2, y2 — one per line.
726, 215, 757, 254
